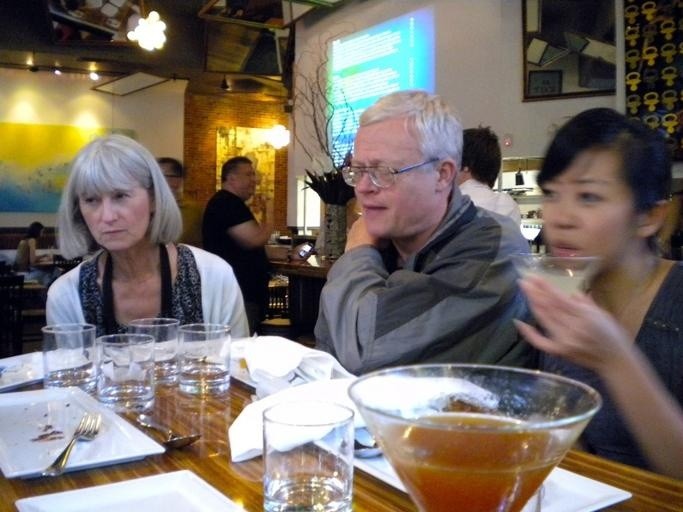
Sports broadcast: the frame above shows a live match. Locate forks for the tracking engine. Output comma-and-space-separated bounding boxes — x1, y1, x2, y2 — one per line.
42, 409, 103, 481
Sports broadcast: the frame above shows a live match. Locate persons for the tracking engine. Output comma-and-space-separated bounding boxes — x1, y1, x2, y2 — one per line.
147, 158, 203, 249
313, 90, 539, 411
457, 129, 521, 224
513, 108, 683, 480
45, 134, 252, 350
203, 157, 312, 336
14, 222, 51, 286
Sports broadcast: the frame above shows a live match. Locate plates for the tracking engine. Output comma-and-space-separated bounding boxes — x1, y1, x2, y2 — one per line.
215, 336, 357, 393
0, 385, 166, 481
14, 467, 250, 511
252, 383, 635, 512
0, 351, 91, 390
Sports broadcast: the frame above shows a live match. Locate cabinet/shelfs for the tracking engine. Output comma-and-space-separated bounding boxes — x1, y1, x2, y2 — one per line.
496, 157, 547, 255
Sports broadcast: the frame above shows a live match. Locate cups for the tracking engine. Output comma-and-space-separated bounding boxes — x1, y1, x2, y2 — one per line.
40, 318, 231, 410
180, 398, 230, 458
510, 257, 600, 306
349, 365, 602, 512
263, 404, 353, 512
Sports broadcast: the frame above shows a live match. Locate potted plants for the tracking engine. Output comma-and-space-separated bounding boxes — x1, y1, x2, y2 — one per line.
297, 167, 356, 263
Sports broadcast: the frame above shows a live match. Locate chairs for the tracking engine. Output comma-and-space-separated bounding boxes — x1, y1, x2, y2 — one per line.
52, 253, 83, 280
0, 261, 24, 360
268, 285, 288, 320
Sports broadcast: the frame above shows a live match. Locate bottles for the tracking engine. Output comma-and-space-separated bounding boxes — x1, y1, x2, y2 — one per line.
528, 237, 546, 254
268, 288, 290, 311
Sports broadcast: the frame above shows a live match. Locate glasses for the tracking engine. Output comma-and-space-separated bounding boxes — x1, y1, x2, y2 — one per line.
341, 157, 446, 188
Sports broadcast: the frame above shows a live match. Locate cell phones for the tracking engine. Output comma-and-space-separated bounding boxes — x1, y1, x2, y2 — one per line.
299, 243, 314, 257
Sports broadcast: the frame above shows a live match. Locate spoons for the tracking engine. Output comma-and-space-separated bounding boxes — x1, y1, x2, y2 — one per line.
136, 413, 201, 449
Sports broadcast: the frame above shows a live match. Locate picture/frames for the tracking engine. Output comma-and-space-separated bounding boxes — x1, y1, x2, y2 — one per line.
521, 0, 616, 102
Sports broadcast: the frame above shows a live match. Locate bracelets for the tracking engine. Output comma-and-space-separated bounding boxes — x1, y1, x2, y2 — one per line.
285, 247, 294, 265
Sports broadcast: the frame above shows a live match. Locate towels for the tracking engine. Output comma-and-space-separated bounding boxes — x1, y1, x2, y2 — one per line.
227, 375, 501, 463
232, 331, 359, 383
